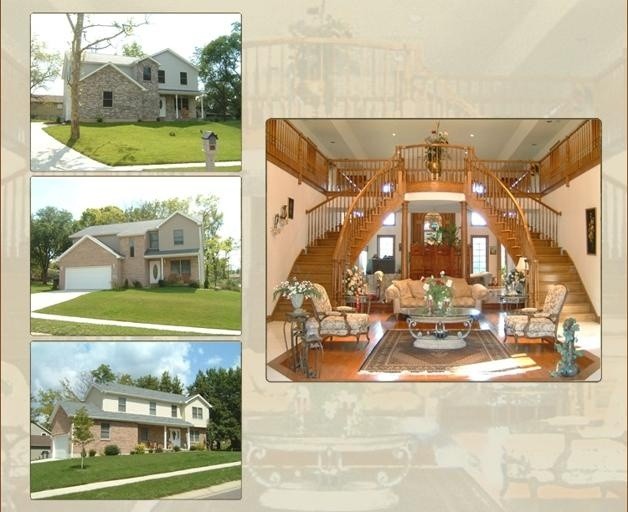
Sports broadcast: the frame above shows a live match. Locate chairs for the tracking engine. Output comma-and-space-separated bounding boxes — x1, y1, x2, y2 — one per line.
504, 283, 568, 350
310, 283, 371, 346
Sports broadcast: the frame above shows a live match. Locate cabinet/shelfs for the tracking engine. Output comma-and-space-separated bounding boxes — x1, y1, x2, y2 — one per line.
409, 240, 470, 280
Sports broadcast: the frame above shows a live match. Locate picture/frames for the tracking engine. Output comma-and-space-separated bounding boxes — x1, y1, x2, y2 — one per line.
585, 207, 596, 255
288, 199, 294, 219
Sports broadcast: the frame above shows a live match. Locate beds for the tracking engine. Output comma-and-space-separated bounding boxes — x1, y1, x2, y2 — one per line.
497, 294, 528, 312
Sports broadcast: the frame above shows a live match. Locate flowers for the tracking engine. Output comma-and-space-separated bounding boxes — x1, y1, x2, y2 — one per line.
272, 277, 322, 300
341, 265, 363, 296
423, 271, 457, 304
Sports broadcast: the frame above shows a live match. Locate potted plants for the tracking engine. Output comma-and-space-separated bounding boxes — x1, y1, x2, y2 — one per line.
96, 112, 104, 122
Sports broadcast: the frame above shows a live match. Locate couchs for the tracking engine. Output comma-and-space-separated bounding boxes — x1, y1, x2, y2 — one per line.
385, 277, 489, 321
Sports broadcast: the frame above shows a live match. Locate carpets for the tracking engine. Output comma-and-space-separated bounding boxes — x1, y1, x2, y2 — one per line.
358, 329, 521, 374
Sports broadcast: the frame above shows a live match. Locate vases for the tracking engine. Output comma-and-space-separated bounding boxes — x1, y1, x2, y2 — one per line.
436, 300, 450, 311
290, 293, 304, 310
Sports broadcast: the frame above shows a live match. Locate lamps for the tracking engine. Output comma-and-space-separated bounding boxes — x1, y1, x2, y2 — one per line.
273, 213, 280, 229
516, 257, 529, 277
279, 203, 287, 220
